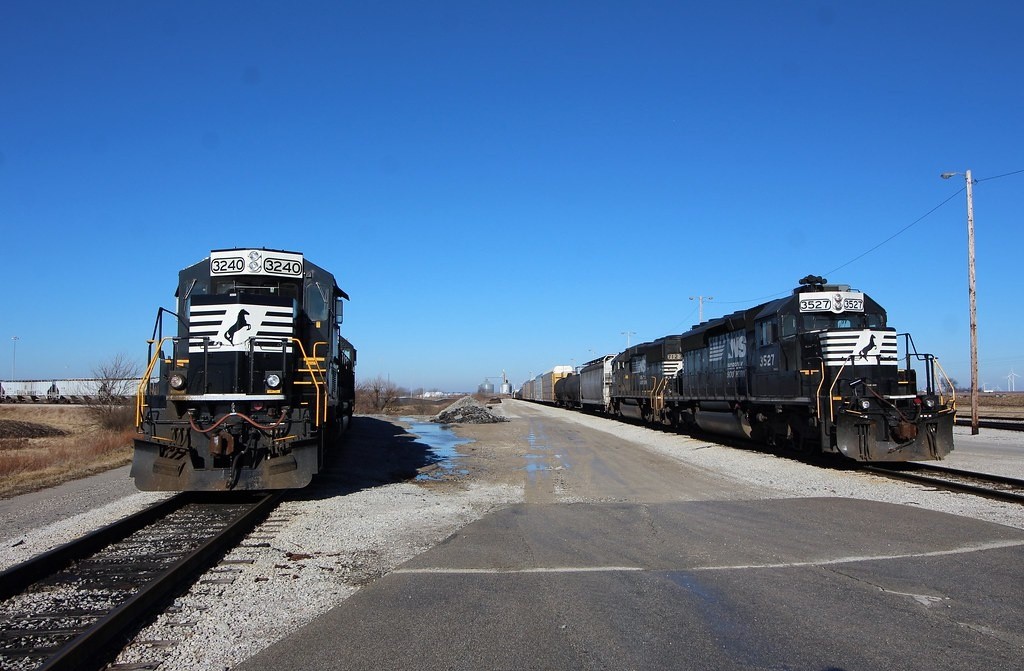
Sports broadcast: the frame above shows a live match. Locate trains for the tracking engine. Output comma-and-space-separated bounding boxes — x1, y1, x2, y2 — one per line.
512, 275, 958, 470
128, 246, 357, 492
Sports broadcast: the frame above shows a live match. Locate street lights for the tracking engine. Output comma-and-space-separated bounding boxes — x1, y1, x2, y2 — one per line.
688, 296, 713, 323
939, 168, 980, 437
621, 332, 637, 349
11, 336, 20, 381
